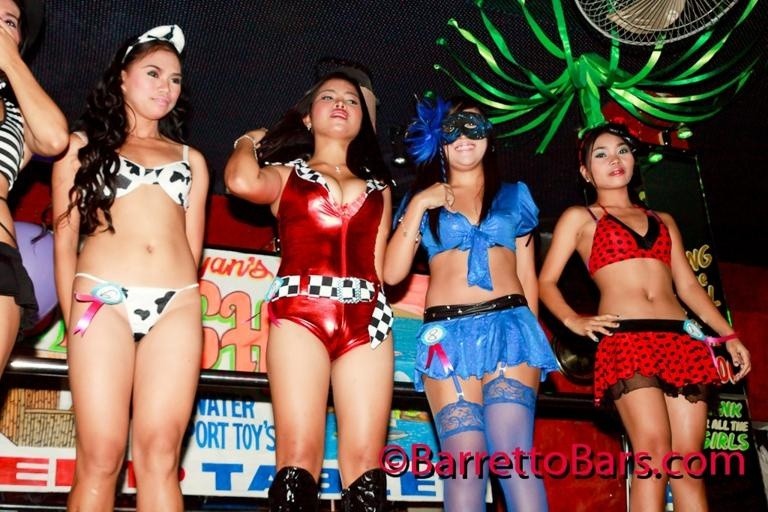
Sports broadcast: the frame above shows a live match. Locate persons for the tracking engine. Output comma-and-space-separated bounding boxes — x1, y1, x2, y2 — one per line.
382, 96, 563, 512
0, 0, 71, 385
226, 73, 393, 511
52, 23, 210, 511
537, 122, 753, 512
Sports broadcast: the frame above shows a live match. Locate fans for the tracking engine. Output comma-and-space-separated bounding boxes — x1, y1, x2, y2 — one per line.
575, 0, 738, 46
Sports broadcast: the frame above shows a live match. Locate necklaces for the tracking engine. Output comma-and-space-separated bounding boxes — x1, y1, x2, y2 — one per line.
334, 164, 342, 176
465, 182, 483, 217
610, 205, 644, 230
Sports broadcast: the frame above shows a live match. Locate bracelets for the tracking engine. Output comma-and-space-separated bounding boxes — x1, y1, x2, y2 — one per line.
234, 135, 260, 162
398, 214, 421, 244
